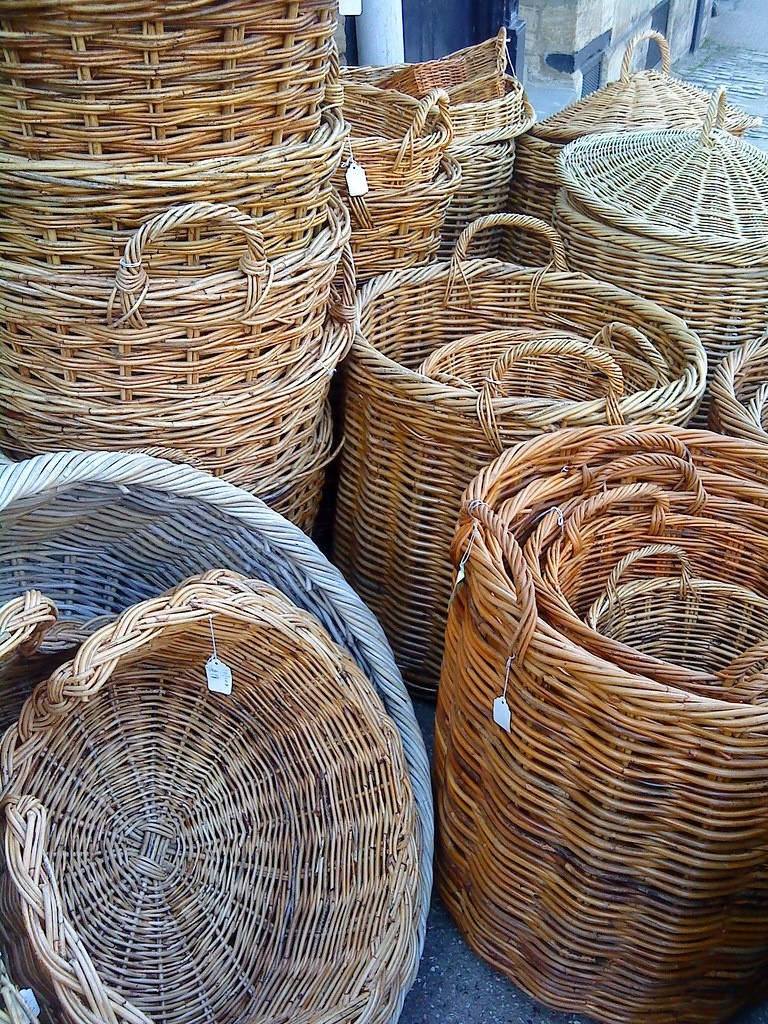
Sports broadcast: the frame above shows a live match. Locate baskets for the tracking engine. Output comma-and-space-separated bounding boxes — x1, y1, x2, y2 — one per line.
0, 0, 768, 1024
